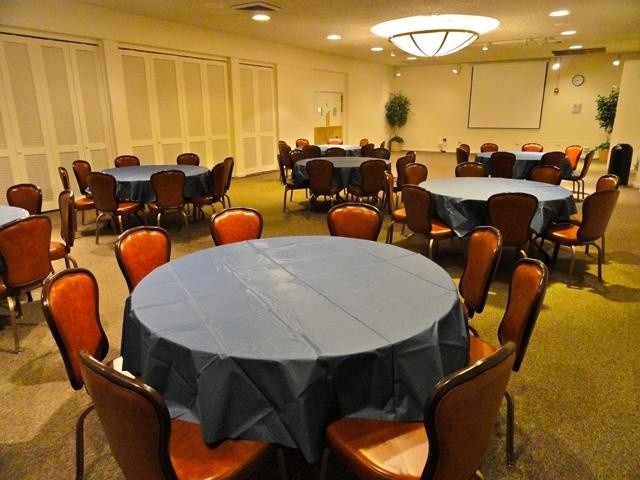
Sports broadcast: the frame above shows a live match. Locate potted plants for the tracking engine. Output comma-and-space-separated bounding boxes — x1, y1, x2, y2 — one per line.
594, 84, 620, 163
384, 88, 412, 153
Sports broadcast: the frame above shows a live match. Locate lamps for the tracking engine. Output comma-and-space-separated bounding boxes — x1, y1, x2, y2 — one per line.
369, 13, 502, 60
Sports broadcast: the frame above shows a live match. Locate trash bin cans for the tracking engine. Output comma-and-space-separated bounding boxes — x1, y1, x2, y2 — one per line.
607, 143, 633, 186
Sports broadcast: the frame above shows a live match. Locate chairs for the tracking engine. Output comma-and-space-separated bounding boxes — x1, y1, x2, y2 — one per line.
57, 153, 235, 245
2, 182, 81, 353
455, 142, 594, 201
41, 201, 549, 480
384, 162, 620, 287
276, 140, 417, 220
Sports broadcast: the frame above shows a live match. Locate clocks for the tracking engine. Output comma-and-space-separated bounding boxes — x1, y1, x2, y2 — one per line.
571, 73, 585, 86
